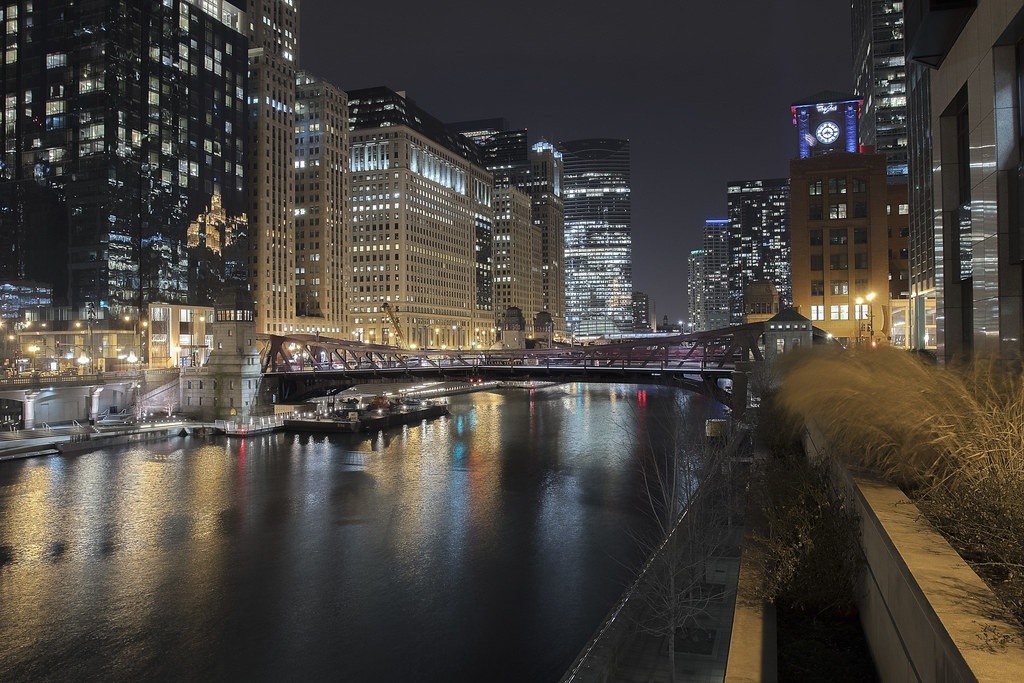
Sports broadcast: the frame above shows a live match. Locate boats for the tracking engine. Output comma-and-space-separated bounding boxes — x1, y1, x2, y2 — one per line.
97, 414, 131, 425
